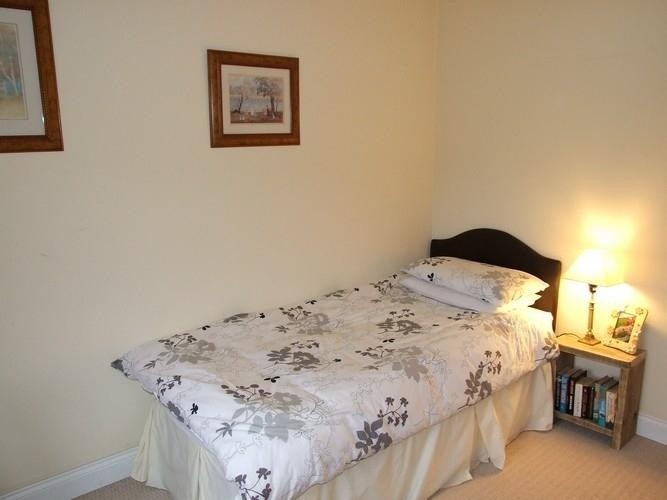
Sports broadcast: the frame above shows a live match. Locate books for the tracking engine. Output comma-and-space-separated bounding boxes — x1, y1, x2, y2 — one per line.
555, 366, 618, 430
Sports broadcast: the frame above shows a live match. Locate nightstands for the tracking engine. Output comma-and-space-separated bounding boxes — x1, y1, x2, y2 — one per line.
551, 333, 647, 451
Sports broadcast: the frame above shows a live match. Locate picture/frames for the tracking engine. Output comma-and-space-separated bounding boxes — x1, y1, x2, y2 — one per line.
204, 47, 302, 148
601, 300, 649, 356
0, 0, 67, 154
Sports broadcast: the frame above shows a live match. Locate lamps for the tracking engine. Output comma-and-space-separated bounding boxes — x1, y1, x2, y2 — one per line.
561, 245, 625, 346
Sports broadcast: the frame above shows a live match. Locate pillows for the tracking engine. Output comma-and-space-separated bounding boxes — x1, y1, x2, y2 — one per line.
398, 255, 550, 308
394, 274, 543, 317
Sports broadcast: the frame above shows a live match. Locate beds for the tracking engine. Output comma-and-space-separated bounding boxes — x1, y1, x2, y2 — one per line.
107, 226, 563, 500
